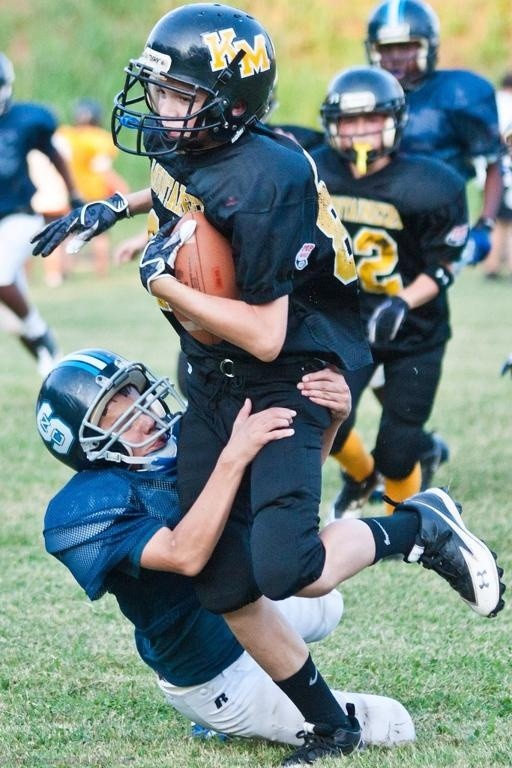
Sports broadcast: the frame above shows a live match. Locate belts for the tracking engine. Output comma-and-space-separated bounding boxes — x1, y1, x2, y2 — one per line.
179, 339, 324, 377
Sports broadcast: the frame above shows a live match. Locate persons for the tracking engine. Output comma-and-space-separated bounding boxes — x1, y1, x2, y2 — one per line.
305, 66, 470, 521
0, 50, 84, 377
359, 0, 504, 501
34, 347, 417, 750
25, 99, 129, 288
31, 0, 505, 768
473, 72, 512, 279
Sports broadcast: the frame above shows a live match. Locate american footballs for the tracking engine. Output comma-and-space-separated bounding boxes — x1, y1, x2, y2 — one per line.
168, 211, 239, 345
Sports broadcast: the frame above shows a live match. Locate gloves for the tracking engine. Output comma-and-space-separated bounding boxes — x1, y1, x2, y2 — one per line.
30, 190, 132, 258
367, 296, 410, 344
459, 219, 493, 266
139, 216, 198, 295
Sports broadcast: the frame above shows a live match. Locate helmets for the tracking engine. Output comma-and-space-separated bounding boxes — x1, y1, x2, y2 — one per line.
135, 3, 277, 127
363, 0, 438, 88
0, 53, 15, 117
36, 347, 188, 474
321, 66, 408, 165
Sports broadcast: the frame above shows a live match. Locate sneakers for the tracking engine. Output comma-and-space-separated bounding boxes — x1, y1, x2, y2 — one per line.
416, 436, 448, 493
382, 487, 505, 618
17, 312, 58, 363
335, 467, 380, 519
275, 703, 361, 768
